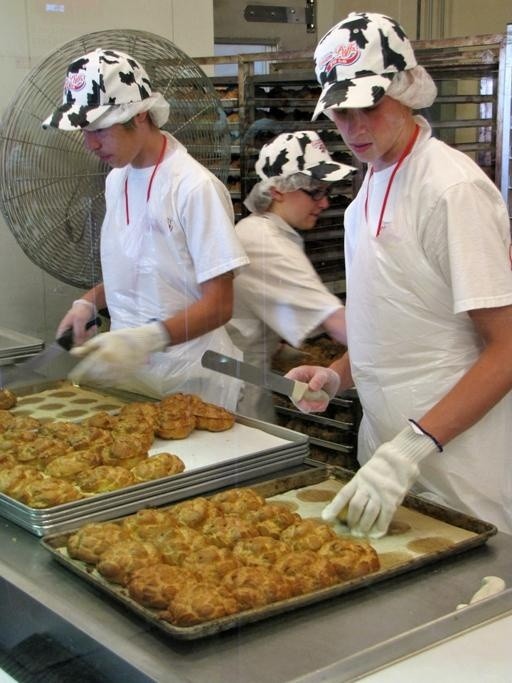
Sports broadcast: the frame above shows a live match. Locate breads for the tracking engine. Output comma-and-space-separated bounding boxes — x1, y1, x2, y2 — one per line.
278, 337, 348, 373
0, 386, 235, 509
67, 487, 379, 626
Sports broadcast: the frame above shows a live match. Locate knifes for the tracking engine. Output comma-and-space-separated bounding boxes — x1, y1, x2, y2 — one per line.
199, 349, 332, 408
0, 314, 103, 387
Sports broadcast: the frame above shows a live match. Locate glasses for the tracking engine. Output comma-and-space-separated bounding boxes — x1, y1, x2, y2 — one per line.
298, 186, 334, 202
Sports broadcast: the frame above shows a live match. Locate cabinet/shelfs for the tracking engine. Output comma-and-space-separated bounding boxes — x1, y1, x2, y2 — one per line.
138, 43, 503, 474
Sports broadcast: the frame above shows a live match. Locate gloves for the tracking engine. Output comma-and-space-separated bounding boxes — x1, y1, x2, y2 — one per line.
68, 318, 172, 381
321, 419, 443, 539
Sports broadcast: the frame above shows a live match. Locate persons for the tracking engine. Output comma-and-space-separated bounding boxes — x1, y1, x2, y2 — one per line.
224, 130, 347, 423
283, 9, 510, 540
40, 47, 247, 416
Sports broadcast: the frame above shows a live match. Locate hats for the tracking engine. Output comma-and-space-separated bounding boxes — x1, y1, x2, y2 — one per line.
42, 49, 152, 132
255, 130, 360, 183
311, 12, 417, 122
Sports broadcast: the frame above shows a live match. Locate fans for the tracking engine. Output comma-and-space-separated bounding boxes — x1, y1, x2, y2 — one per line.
1, 28, 232, 317
